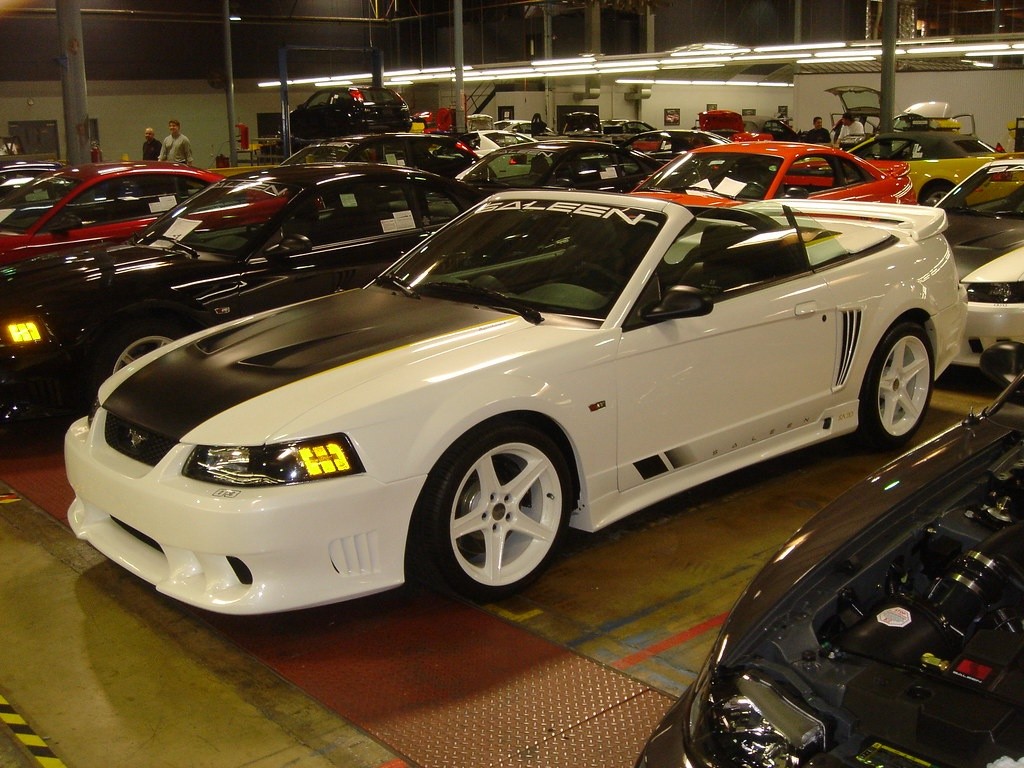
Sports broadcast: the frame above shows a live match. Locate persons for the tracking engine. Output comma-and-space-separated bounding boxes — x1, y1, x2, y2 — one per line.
436, 101, 468, 134
143, 127, 162, 161
835, 112, 864, 144
802, 117, 831, 143
158, 120, 193, 166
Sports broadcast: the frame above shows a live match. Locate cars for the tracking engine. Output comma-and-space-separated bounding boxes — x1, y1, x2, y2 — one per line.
933, 160, 1024, 367
279, 85, 1024, 214
635, 340, 1023, 768
289, 85, 412, 139
64, 190, 968, 614
0, 161, 490, 448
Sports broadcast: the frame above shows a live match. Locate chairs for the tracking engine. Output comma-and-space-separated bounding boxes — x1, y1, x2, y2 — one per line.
687, 222, 756, 285
553, 215, 624, 278
